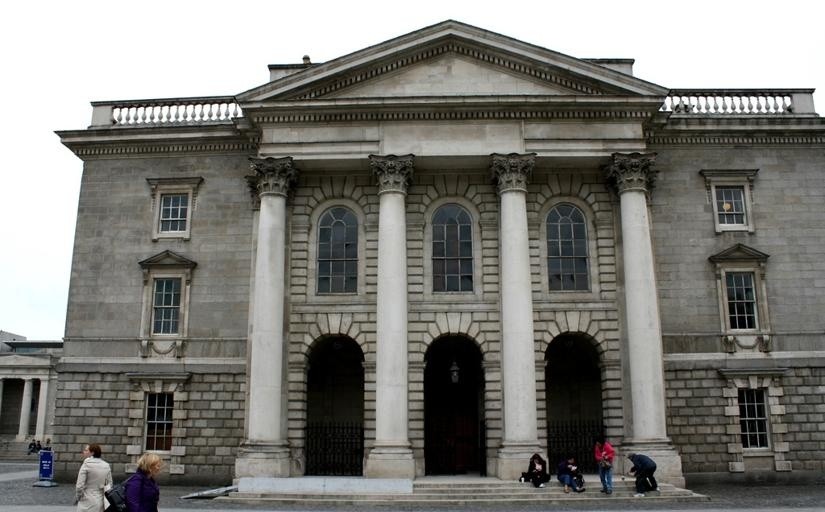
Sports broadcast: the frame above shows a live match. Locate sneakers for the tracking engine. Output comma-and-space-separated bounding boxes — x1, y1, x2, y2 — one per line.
533, 480, 612, 495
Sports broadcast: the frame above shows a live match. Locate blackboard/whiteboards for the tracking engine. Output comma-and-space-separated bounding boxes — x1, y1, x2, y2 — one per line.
39, 450, 54, 480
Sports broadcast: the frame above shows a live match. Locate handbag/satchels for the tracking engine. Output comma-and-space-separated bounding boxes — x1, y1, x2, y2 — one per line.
600, 455, 612, 469
518, 472, 530, 482
103, 475, 137, 512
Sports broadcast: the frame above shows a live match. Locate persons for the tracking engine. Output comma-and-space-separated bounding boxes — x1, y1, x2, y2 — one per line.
627, 452, 660, 498
122, 452, 163, 511
35, 440, 42, 450
42, 437, 52, 451
527, 453, 551, 488
26, 438, 38, 455
557, 455, 587, 493
71, 442, 114, 512
592, 433, 616, 494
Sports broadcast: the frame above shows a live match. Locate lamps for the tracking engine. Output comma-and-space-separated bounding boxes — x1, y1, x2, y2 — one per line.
448, 362, 460, 383
